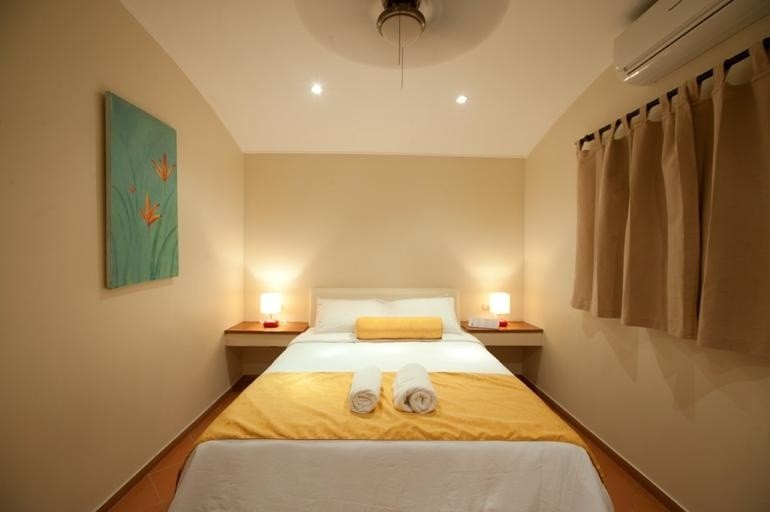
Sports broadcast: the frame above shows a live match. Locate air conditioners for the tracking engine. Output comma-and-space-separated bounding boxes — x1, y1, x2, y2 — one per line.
614, 0, 770, 86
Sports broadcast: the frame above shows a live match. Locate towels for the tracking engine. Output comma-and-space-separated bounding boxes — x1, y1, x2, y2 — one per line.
392, 362, 438, 414
348, 367, 381, 414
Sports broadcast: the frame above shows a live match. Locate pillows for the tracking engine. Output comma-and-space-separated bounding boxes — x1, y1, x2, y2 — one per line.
313, 297, 463, 339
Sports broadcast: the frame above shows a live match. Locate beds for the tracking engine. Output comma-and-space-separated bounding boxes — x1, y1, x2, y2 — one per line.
168, 289, 615, 512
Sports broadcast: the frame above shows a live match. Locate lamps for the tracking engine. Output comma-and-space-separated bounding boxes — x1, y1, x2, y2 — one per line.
260, 293, 281, 328
488, 292, 510, 327
294, 0, 511, 89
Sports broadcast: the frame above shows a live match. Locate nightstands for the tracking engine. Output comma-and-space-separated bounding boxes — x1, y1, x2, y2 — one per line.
460, 321, 544, 346
224, 321, 308, 347
